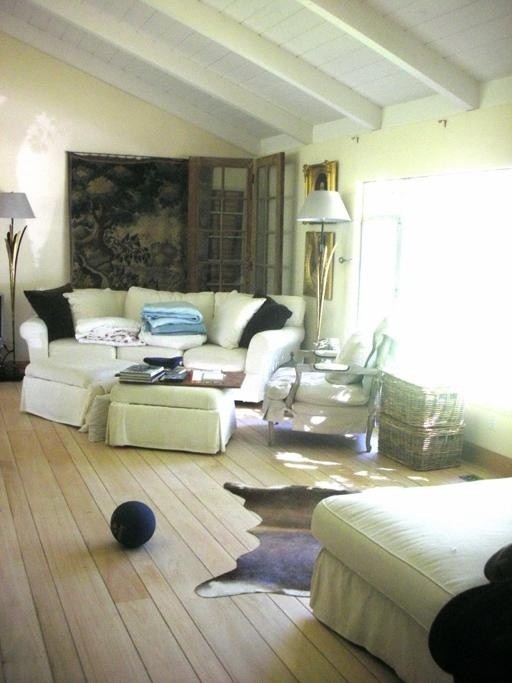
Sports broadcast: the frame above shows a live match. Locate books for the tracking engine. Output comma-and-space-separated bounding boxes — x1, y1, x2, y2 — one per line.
159, 370, 190, 382
118, 363, 165, 377
117, 371, 166, 383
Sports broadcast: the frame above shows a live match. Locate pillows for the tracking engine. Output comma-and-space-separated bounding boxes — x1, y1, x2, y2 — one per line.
326, 362, 363, 385
239, 291, 293, 349
123, 284, 214, 334
22, 283, 74, 343
63, 287, 121, 334
208, 289, 267, 349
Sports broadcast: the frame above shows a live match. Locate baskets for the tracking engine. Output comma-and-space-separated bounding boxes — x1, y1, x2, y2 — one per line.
378, 366, 467, 431
376, 412, 467, 472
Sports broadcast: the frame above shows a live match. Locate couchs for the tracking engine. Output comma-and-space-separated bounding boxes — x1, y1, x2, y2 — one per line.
19, 288, 306, 408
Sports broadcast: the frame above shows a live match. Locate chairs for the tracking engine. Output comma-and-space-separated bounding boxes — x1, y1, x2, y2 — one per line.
261, 317, 391, 453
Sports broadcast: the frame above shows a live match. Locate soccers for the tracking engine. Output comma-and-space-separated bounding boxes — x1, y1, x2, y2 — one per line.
110, 500, 155, 547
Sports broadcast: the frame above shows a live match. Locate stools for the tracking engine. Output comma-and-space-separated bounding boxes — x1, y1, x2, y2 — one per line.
19, 351, 140, 428
104, 385, 237, 454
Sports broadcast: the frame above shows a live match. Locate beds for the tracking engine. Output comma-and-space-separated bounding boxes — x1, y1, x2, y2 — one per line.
310, 476, 511, 682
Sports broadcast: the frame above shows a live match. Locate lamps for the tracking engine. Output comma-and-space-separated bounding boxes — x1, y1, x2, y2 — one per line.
1, 190, 36, 382
294, 188, 352, 347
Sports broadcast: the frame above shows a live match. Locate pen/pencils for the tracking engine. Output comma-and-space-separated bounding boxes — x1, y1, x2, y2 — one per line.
179, 368, 187, 375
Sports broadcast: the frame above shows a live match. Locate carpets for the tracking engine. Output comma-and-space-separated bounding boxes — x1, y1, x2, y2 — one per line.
195, 482, 363, 597
